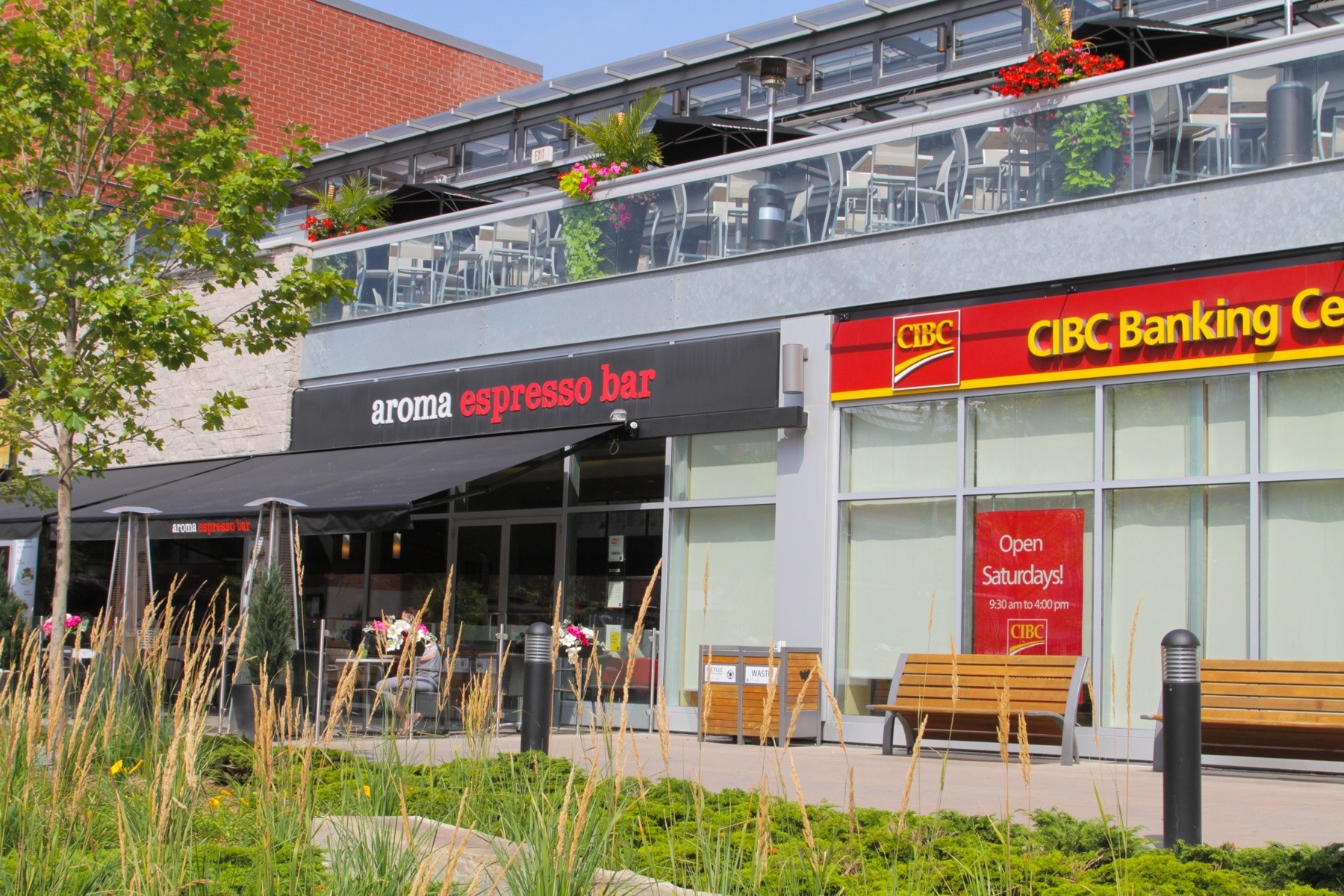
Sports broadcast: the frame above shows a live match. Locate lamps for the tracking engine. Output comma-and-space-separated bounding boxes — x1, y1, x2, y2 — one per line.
781, 343, 809, 396
447, 146, 459, 168
560, 22, 949, 142
393, 529, 402, 559
340, 533, 351, 560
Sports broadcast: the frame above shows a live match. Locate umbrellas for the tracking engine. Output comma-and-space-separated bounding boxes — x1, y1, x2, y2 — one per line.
645, 108, 820, 184
1021, 16, 1268, 193
370, 180, 504, 273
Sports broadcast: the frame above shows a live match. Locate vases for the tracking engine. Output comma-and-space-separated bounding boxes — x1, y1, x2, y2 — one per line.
365, 633, 426, 656
45, 630, 88, 646
326, 253, 356, 326
1045, 131, 1121, 203
559, 645, 603, 659
591, 202, 648, 274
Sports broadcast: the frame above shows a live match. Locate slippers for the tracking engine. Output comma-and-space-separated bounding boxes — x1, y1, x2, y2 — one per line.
401, 712, 423, 735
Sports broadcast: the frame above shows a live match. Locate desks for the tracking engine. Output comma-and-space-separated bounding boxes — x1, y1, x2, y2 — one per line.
334, 658, 400, 736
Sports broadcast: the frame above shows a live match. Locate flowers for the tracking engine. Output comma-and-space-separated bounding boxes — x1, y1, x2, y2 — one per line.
991, 0, 1135, 197
42, 615, 90, 638
362, 614, 438, 651
297, 163, 399, 278
554, 85, 668, 283
551, 617, 612, 665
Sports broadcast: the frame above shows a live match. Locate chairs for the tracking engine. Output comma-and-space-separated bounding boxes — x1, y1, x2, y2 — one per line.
346, 211, 569, 317
293, 648, 597, 738
640, 64, 1344, 267
63, 643, 236, 716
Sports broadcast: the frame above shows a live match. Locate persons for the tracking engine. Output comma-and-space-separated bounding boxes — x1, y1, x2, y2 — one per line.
376, 605, 443, 735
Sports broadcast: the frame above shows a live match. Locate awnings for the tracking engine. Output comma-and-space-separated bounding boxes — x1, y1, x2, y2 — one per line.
0, 424, 623, 543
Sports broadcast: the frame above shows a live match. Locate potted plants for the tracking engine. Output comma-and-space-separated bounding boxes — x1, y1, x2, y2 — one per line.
230, 558, 298, 742
0, 549, 31, 707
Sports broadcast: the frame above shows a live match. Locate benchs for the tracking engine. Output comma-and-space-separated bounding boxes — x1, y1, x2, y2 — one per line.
1141, 659, 1344, 762
866, 653, 1089, 766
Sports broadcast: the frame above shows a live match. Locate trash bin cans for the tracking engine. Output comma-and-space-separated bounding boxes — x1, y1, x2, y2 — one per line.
747, 182, 788, 253
1267, 82, 1313, 167
